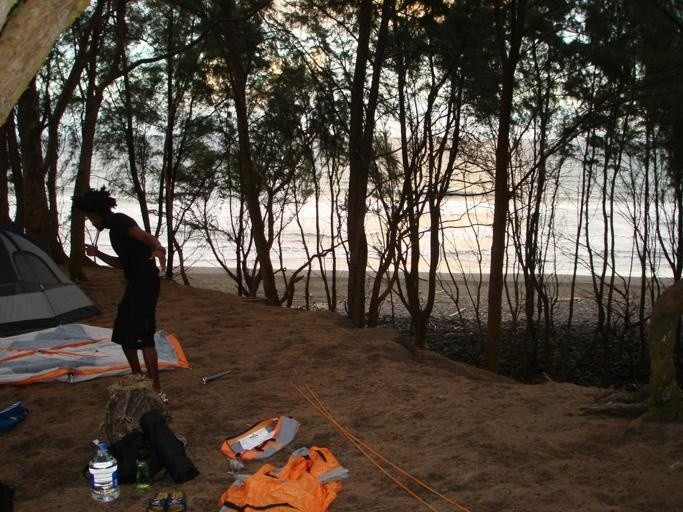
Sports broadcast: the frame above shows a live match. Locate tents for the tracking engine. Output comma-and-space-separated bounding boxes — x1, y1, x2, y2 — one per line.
0, 228, 103, 339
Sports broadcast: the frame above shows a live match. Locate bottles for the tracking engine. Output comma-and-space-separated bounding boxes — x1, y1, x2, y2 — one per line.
135, 458, 150, 493
88, 443, 121, 503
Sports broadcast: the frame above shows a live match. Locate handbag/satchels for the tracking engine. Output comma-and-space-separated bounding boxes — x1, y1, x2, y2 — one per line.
139, 408, 199, 483
84, 428, 165, 484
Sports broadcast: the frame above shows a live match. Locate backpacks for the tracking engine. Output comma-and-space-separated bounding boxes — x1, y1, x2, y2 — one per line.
99, 374, 169, 443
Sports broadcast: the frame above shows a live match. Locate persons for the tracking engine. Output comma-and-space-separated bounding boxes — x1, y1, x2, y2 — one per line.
82, 190, 168, 403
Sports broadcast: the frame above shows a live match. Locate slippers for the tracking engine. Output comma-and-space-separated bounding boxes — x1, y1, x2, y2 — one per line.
147, 491, 169, 512
167, 490, 187, 512
